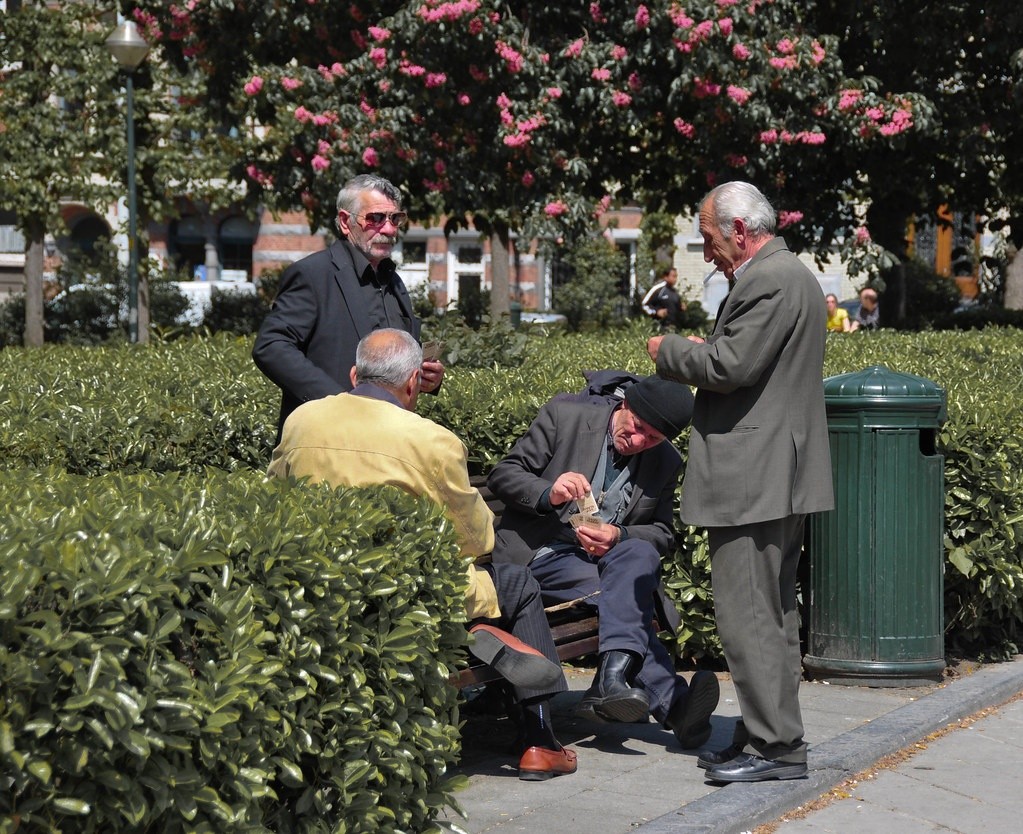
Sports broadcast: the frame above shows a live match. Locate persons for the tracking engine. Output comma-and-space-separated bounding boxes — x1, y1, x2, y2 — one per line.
648, 181, 835, 783
253, 175, 444, 446
484, 376, 720, 749
266, 327, 577, 781
642, 266, 686, 335
826, 287, 880, 333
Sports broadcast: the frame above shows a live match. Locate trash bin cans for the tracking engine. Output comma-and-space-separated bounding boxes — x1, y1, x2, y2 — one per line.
510, 302, 524, 326
802, 368, 947, 689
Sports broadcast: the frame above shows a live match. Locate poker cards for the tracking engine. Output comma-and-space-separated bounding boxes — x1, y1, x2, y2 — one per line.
568, 491, 603, 532
421, 337, 449, 363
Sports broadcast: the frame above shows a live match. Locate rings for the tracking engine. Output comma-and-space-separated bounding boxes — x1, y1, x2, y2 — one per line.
590, 546, 595, 553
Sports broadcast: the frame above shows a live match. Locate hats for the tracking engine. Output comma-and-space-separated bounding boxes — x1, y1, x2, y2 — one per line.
624, 374, 695, 441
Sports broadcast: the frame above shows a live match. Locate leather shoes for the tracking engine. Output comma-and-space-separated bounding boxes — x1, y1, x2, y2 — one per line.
517, 740, 578, 781
704, 753, 808, 781
696, 742, 745, 768
466, 623, 563, 691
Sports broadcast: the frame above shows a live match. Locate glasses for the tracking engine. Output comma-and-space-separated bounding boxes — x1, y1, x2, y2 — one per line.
349, 211, 409, 227
826, 301, 835, 303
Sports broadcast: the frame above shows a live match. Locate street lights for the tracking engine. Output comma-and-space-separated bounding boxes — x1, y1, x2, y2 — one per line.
104, 19, 152, 345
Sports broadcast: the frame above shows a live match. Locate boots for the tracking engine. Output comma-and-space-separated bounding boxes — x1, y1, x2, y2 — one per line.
665, 670, 721, 750
576, 650, 651, 726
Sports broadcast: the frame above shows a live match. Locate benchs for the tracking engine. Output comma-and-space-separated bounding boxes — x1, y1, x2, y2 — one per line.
448, 473, 665, 689
